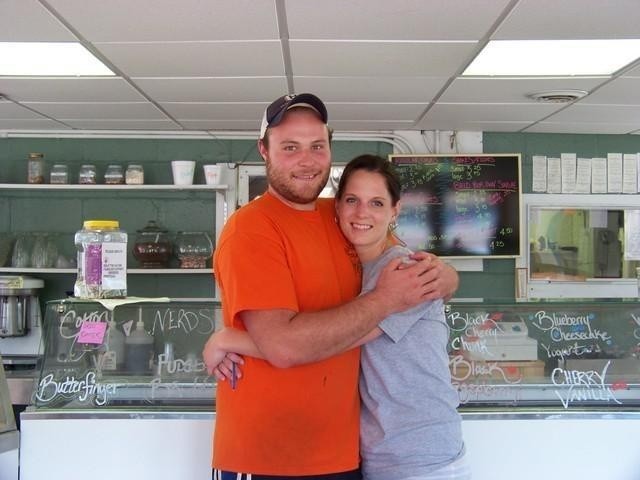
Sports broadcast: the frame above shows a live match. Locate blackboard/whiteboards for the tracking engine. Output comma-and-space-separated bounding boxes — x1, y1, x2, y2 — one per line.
390, 154, 522, 259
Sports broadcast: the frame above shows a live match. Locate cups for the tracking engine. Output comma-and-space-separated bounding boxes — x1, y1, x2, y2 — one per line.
170, 160, 196, 185
202, 164, 222, 185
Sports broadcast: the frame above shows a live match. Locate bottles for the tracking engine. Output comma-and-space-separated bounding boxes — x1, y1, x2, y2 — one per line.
125, 164, 145, 185
103, 164, 124, 185
10, 230, 63, 269
97, 306, 155, 371
49, 161, 69, 184
77, 162, 97, 184
27, 152, 44, 184
72, 218, 129, 300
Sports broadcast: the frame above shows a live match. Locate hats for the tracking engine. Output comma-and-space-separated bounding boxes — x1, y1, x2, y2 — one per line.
260, 93, 327, 140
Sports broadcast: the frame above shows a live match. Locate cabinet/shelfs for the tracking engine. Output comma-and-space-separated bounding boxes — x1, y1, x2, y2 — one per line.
0, 182, 229, 270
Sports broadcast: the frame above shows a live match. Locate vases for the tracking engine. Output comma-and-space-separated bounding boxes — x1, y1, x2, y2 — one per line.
172, 160, 220, 186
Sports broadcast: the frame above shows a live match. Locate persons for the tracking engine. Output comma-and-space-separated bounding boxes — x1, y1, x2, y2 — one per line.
210, 94, 463, 480
202, 154, 469, 480
